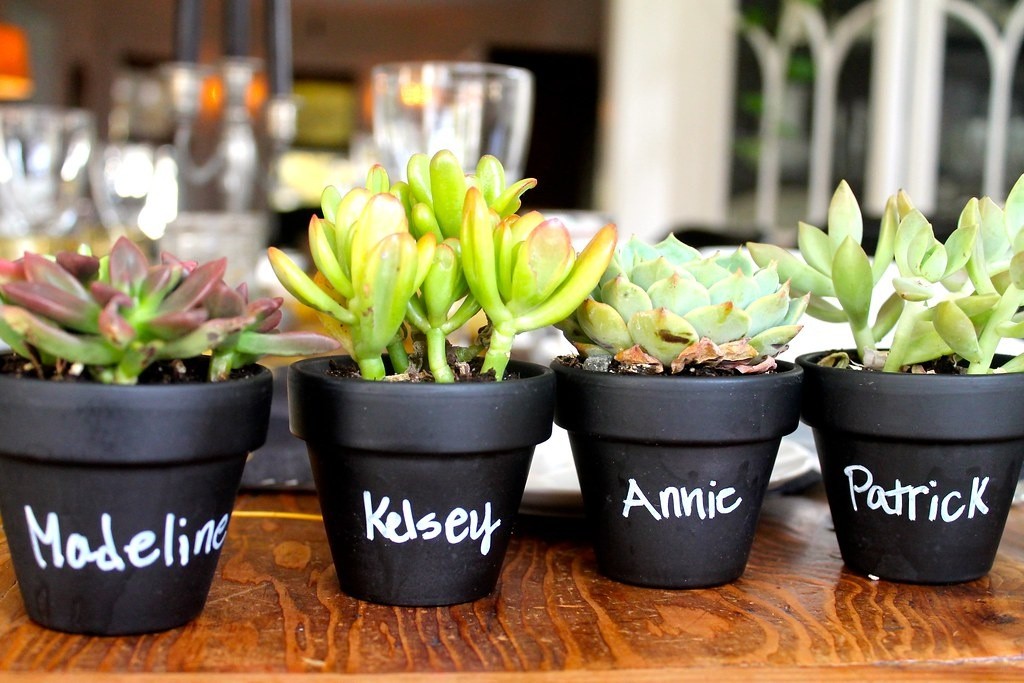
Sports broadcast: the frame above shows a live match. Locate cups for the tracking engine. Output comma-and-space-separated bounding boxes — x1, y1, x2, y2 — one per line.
160, 212, 269, 298
0, 102, 184, 265
368, 61, 534, 206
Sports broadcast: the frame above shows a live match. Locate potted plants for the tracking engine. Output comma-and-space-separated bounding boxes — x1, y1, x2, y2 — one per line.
268, 148, 618, 608
551, 231, 812, 591
0, 236, 340, 634
747, 173, 1024, 584
